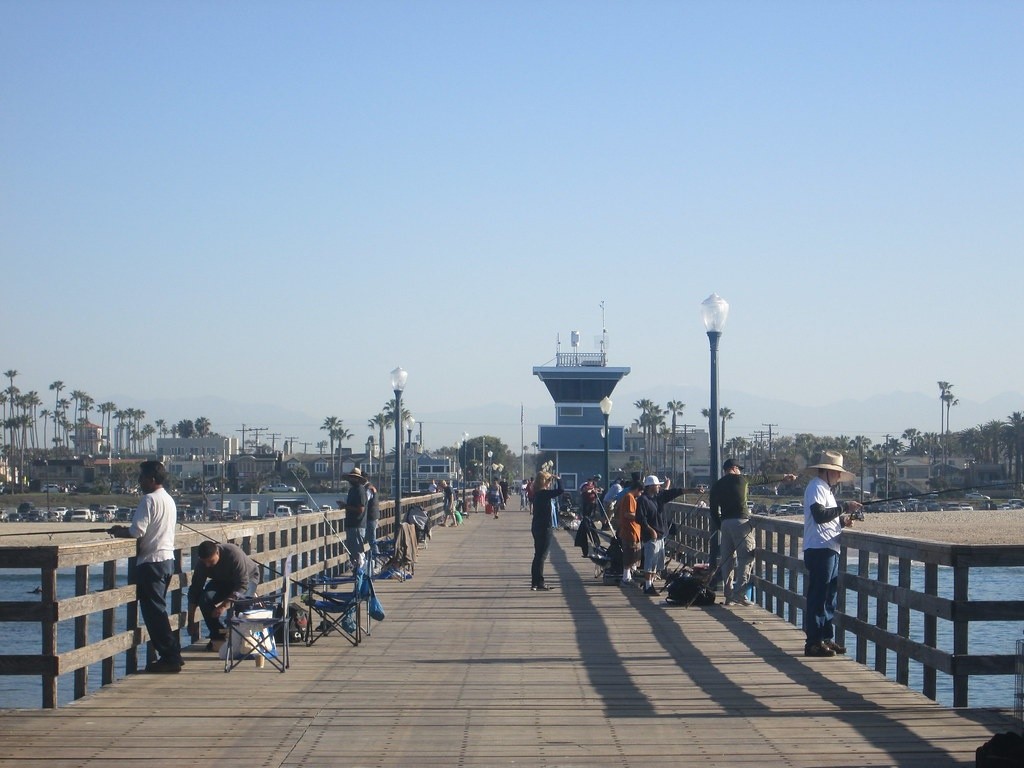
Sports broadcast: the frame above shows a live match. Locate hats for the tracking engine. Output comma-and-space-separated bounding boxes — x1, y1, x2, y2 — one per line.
645, 475, 664, 486
348, 467, 367, 484
724, 459, 745, 471
808, 450, 855, 482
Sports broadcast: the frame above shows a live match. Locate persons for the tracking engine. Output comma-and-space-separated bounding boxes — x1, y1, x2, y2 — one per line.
336, 467, 381, 577
106, 460, 185, 674
472, 478, 509, 518
520, 477, 534, 512
619, 475, 671, 588
803, 451, 864, 655
636, 475, 705, 596
428, 479, 458, 527
187, 541, 260, 641
531, 470, 565, 591
710, 459, 798, 606
579, 473, 645, 529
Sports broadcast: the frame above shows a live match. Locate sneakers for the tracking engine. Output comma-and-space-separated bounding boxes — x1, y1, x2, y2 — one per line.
805, 639, 846, 657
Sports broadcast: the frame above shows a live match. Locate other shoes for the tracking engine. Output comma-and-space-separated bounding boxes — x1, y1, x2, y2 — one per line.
532, 584, 555, 591
146, 660, 181, 674
619, 578, 639, 587
725, 592, 754, 606
449, 525, 457, 528
438, 523, 448, 527
644, 583, 662, 597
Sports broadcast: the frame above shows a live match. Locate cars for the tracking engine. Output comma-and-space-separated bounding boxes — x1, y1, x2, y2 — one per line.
262, 505, 333, 519
746, 500, 805, 516
0, 502, 243, 523
696, 484, 710, 488
861, 492, 1024, 511
40, 484, 69, 493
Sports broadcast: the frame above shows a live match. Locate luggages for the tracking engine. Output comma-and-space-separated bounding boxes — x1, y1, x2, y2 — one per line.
455, 511, 463, 525
485, 504, 492, 514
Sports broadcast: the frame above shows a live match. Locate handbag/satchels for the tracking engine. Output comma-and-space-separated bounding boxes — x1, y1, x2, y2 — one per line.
500, 503, 505, 510
274, 602, 309, 643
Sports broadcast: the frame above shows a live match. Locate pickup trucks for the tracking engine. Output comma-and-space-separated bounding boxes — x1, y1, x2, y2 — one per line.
265, 484, 296, 493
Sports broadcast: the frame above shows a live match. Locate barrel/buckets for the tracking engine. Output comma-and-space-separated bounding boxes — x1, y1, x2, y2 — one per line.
693, 563, 709, 579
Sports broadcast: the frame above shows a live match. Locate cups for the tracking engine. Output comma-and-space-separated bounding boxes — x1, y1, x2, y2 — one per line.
255, 652, 265, 669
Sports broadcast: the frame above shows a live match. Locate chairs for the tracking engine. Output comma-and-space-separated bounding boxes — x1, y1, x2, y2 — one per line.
222, 577, 290, 673
579, 511, 617, 578
371, 505, 429, 582
304, 549, 374, 646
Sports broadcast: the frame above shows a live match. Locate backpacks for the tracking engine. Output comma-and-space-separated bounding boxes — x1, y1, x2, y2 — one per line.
667, 577, 716, 605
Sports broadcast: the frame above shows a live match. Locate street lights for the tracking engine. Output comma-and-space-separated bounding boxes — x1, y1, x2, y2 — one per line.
406, 416, 415, 499
390, 366, 408, 544
702, 294, 729, 577
461, 432, 469, 514
488, 451, 493, 485
454, 441, 459, 487
599, 396, 613, 491
542, 460, 554, 484
